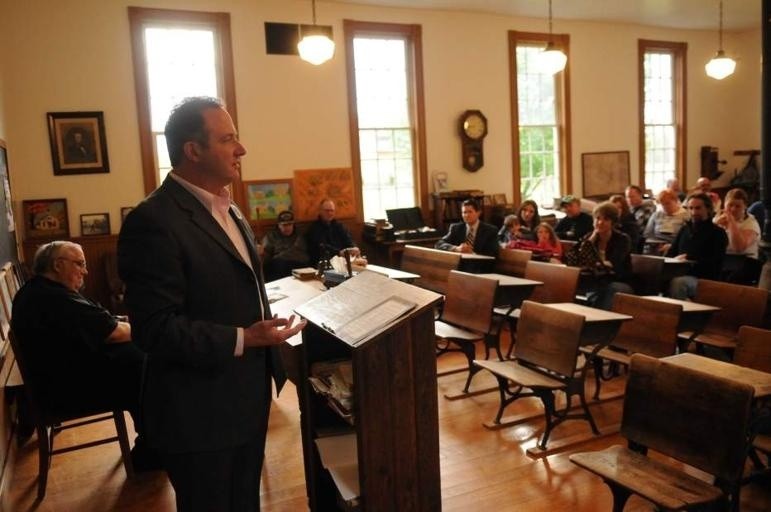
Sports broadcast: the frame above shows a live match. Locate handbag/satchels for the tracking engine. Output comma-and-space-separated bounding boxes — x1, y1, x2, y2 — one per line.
566, 241, 605, 272
506, 238, 553, 261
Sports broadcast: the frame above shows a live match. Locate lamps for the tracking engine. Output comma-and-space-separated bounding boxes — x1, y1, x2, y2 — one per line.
535, 0, 567, 74
297, 0, 335, 64
704, 1, 736, 80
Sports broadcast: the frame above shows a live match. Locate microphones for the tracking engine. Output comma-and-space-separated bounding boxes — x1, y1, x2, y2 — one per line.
343, 248, 352, 277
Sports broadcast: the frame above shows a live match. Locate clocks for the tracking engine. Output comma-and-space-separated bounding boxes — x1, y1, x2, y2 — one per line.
459, 110, 487, 171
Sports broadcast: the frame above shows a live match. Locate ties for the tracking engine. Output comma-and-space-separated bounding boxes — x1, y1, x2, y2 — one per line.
466, 226, 474, 246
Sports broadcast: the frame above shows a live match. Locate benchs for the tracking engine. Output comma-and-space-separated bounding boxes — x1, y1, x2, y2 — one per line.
434, 249, 751, 403
469, 280, 753, 461
403, 237, 669, 320
0, 261, 61, 448
568, 326, 753, 511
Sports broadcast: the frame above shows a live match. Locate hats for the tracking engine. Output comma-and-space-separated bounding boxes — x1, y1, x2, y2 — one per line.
560, 195, 579, 207
278, 211, 294, 225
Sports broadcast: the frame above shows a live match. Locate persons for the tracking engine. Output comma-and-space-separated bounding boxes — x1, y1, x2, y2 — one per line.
300, 195, 360, 259
256, 209, 313, 283
7, 240, 169, 472
609, 178, 761, 298
565, 202, 638, 310
436, 199, 499, 274
497, 200, 562, 264
554, 195, 594, 242
115, 98, 308, 510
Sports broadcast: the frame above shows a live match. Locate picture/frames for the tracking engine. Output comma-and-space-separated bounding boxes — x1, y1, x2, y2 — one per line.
23, 199, 69, 239
242, 178, 296, 224
80, 212, 110, 236
47, 111, 110, 175
581, 150, 631, 198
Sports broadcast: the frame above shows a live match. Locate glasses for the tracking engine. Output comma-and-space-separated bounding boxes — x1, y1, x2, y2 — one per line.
58, 257, 85, 269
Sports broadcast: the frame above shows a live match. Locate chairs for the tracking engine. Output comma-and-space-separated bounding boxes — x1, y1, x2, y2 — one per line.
8, 329, 137, 499
